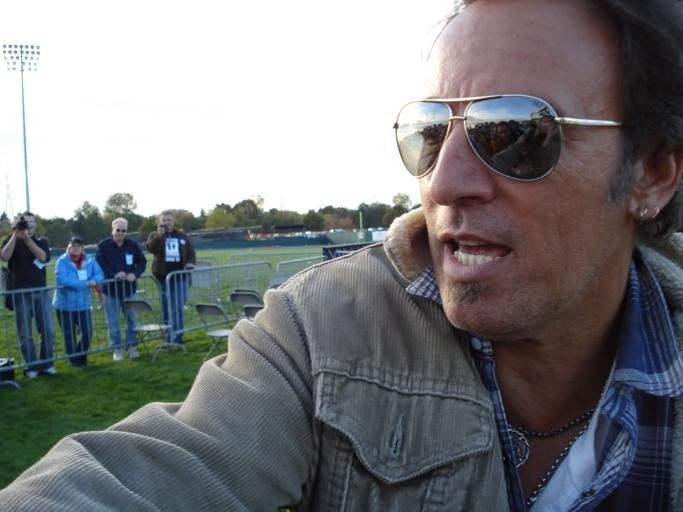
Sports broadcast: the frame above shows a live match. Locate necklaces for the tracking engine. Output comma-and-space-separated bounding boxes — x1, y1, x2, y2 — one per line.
504, 402, 594, 509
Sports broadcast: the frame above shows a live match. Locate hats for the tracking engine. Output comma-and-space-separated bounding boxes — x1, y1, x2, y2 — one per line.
71, 235, 81, 244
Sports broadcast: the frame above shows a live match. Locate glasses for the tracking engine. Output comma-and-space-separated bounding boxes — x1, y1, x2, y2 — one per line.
394, 93, 622, 183
113, 229, 126, 232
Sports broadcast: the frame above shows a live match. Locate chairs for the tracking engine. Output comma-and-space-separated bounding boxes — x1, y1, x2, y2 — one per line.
123, 282, 286, 364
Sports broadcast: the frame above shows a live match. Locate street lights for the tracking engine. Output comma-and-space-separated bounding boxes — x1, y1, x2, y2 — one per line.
3, 45, 39, 212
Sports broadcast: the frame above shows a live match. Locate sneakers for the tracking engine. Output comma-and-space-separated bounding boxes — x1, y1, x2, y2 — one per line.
112, 345, 142, 361
23, 367, 59, 378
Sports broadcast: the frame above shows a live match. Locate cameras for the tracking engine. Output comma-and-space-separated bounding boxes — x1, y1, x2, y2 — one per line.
160, 224, 166, 228
16, 216, 28, 231
540, 124, 549, 131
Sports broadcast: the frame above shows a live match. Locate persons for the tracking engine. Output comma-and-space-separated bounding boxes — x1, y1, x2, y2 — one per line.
94, 217, 148, 361
0, 1, 683, 512
466, 113, 559, 179
145, 209, 196, 350
49, 235, 105, 367
0, 212, 59, 379
416, 122, 446, 148
